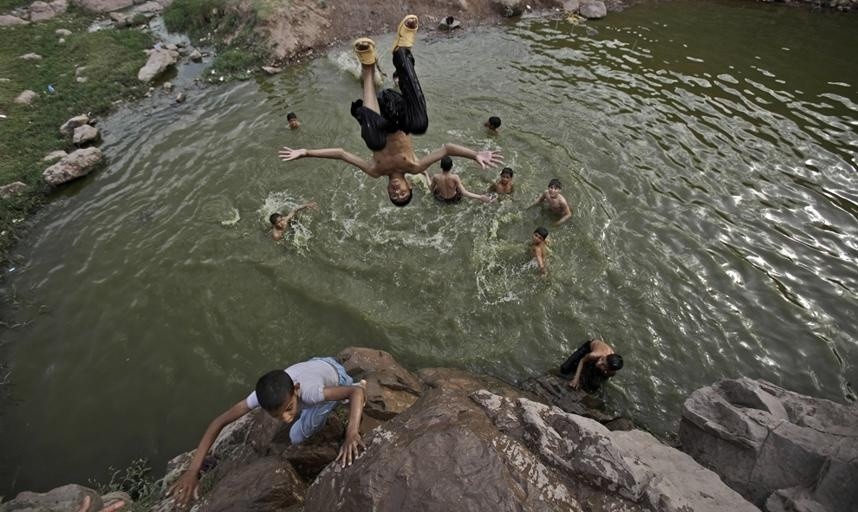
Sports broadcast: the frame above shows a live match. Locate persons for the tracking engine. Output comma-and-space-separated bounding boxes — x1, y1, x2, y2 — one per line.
165, 357, 367, 509
487, 167, 514, 194
285, 112, 300, 130
532, 227, 551, 277
421, 157, 490, 204
560, 338, 624, 390
269, 201, 316, 240
525, 179, 573, 226
484, 115, 502, 138
278, 14, 506, 208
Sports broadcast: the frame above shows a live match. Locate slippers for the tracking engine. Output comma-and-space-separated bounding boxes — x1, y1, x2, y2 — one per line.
388, 14, 420, 55
356, 37, 378, 69
